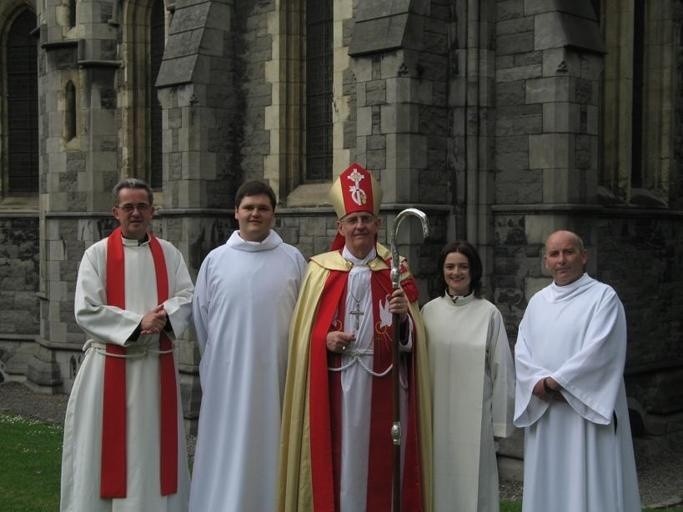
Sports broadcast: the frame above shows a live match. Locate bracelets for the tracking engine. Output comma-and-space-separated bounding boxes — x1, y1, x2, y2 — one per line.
543, 377, 556, 394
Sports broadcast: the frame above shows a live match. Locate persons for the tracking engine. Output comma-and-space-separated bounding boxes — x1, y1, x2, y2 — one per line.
276, 161, 436, 512
187, 178, 308, 512
514, 229, 643, 512
420, 239, 514, 511
59, 177, 193, 512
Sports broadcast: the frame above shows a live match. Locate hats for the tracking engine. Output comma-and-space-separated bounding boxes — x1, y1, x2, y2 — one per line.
329, 163, 383, 220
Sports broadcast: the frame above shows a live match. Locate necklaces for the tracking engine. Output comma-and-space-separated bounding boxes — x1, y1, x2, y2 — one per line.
345, 281, 369, 330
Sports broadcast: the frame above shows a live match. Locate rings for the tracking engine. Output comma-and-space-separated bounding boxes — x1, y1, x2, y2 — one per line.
342, 344, 346, 351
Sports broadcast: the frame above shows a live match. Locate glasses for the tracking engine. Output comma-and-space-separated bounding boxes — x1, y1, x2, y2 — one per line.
340, 215, 374, 224
117, 204, 149, 212
